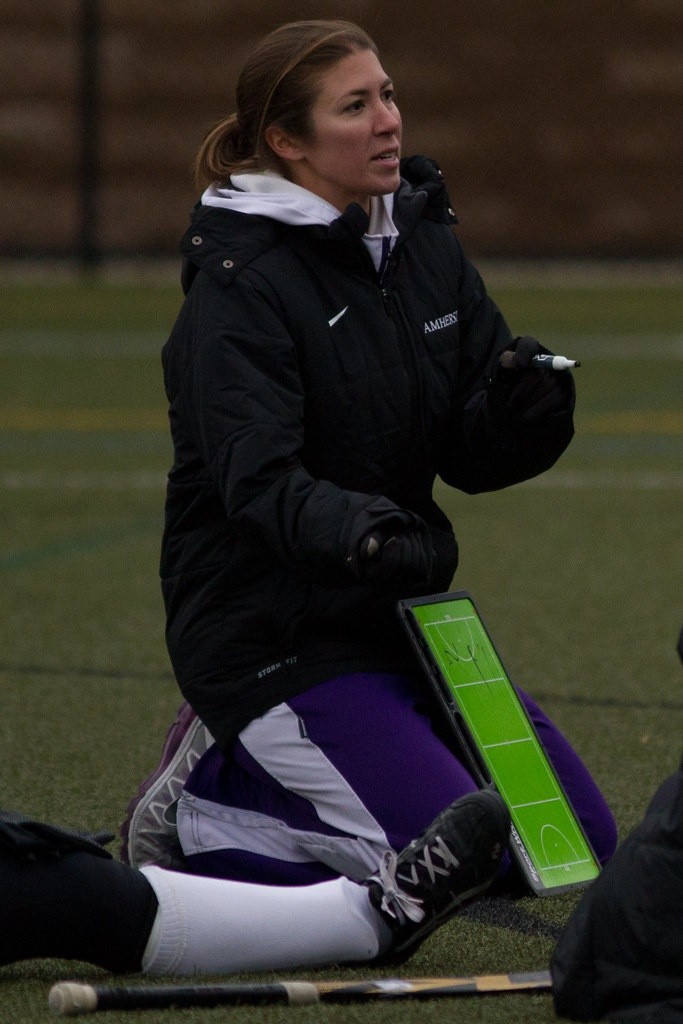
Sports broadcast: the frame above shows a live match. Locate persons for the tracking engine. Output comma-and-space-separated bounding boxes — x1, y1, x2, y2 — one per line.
550, 634, 683, 1023
118, 21, 616, 903
0, 790, 511, 974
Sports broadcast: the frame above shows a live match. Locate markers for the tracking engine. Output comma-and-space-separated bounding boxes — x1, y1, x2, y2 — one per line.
500, 351, 581, 371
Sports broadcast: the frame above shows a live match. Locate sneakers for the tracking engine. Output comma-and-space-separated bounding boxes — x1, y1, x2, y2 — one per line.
349, 790, 511, 970
122, 699, 221, 870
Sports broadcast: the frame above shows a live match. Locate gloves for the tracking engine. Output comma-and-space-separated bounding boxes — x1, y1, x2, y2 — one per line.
358, 514, 439, 595
500, 334, 577, 432
